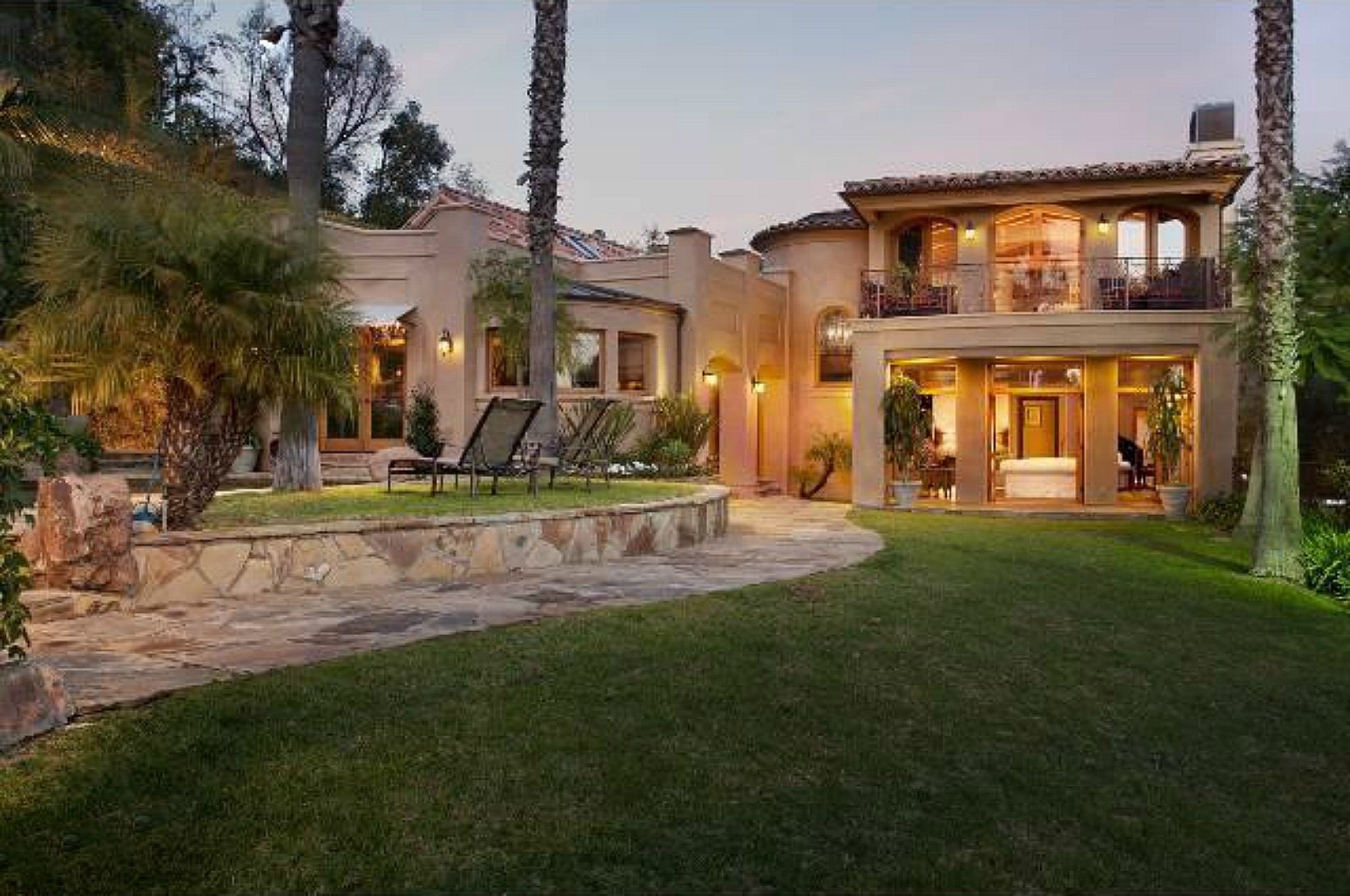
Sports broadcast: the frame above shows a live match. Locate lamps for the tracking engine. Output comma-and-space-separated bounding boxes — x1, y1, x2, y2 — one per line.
1097, 214, 1110, 233
752, 378, 765, 393
702, 370, 716, 386
438, 329, 452, 357
965, 223, 976, 238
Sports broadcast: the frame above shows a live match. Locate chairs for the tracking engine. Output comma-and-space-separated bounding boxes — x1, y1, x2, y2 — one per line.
388, 396, 622, 499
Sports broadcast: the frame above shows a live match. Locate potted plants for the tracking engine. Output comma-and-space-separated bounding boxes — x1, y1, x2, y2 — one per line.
878, 374, 927, 505
1143, 367, 1197, 511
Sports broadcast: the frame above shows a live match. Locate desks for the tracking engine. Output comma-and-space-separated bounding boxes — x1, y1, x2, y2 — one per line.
920, 468, 954, 499
